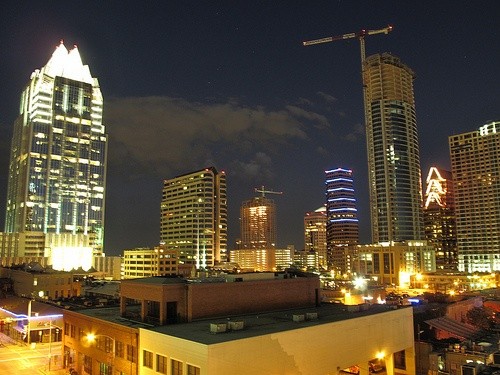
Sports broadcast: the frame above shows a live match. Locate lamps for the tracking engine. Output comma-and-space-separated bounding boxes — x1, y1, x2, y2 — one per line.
376, 350, 384, 359
